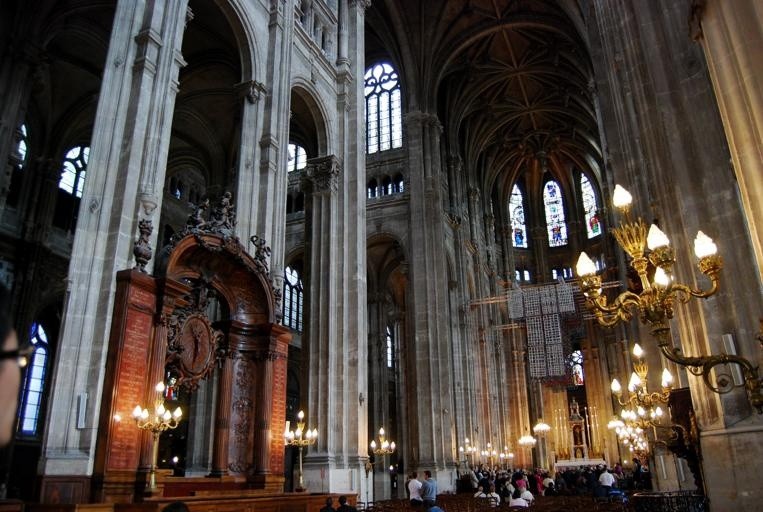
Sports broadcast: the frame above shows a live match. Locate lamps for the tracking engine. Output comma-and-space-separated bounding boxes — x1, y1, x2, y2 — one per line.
284, 410, 319, 493
130, 380, 183, 495
457, 420, 552, 469
365, 427, 396, 478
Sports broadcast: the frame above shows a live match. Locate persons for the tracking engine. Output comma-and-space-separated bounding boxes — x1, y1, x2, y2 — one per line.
250, 235, 272, 265
419, 470, 436, 509
405, 472, 413, 505
319, 497, 336, 512
426, 500, 444, 512
407, 472, 422, 512
336, 496, 358, 512
190, 190, 237, 229
466, 458, 649, 508
